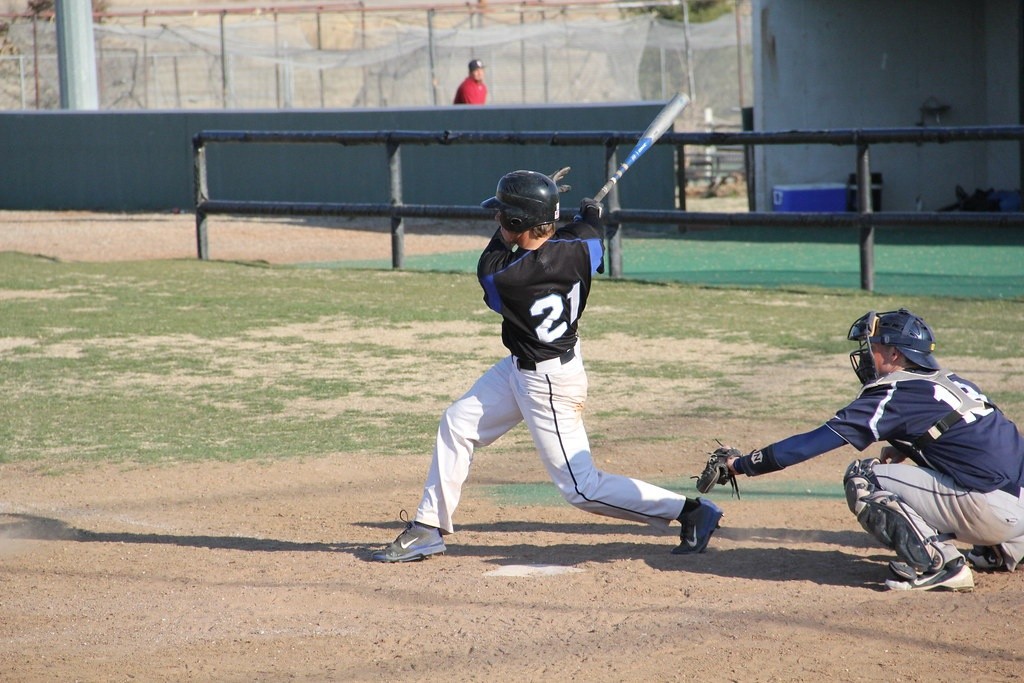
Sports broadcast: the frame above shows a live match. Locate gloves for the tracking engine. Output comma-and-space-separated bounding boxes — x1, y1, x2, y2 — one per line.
547, 166, 572, 193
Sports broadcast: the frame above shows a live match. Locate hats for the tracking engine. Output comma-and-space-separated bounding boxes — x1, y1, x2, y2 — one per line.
468, 59, 484, 71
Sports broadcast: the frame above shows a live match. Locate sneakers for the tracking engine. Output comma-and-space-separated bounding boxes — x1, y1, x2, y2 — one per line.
884, 556, 975, 594
671, 496, 725, 555
965, 545, 1013, 571
371, 509, 447, 562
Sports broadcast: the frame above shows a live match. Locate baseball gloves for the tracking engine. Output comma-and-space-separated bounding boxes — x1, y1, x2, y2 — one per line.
696, 447, 744, 494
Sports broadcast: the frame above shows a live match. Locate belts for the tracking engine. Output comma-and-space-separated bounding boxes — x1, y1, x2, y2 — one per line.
509, 344, 576, 372
1000, 481, 1020, 498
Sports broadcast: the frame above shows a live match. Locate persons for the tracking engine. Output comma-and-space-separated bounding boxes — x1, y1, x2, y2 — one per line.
453, 59, 488, 106
372, 166, 724, 562
697, 308, 1023, 593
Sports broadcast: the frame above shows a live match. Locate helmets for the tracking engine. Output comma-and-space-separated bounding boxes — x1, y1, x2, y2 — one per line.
872, 308, 942, 369
479, 170, 560, 232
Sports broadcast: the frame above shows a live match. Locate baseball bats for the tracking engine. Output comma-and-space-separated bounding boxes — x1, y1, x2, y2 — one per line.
573, 90, 691, 223
